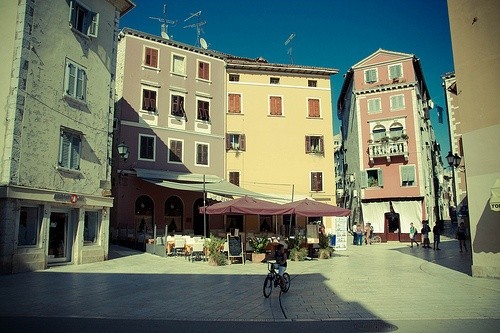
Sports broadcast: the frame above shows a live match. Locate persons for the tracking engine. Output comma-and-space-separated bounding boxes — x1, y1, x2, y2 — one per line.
433, 221, 441, 250
457, 222, 467, 253
421, 220, 432, 248
353, 222, 374, 246
263, 243, 287, 293
409, 222, 419, 248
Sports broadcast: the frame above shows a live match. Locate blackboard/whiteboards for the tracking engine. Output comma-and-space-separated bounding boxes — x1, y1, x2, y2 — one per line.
227, 233, 243, 257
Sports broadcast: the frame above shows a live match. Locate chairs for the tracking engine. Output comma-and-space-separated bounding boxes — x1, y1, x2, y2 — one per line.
156, 232, 297, 262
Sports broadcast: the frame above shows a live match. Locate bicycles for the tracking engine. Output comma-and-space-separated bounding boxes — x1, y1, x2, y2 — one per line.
262, 261, 291, 298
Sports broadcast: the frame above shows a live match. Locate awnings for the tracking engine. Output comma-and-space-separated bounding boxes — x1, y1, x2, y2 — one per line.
489, 179, 500, 211
132, 168, 291, 200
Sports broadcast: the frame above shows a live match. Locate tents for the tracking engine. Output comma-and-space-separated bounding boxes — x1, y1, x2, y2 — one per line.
198, 195, 351, 244
